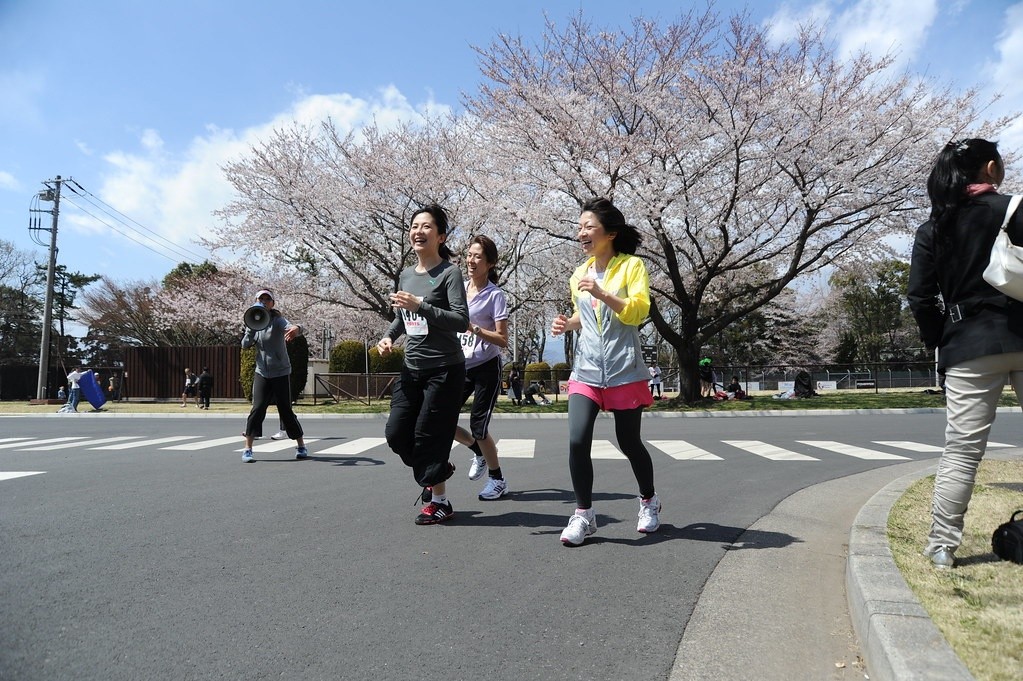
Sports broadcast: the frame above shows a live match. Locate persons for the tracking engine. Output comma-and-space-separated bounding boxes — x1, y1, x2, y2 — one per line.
522, 381, 548, 405
794, 370, 811, 397
905, 137, 1023, 569
509, 362, 521, 406
647, 363, 660, 398
699, 362, 741, 399
243, 413, 290, 440
66, 365, 91, 412
551, 197, 660, 545
198, 367, 213, 409
94, 373, 102, 388
453, 234, 508, 500
58, 387, 65, 399
180, 368, 199, 408
375, 204, 469, 525
241, 289, 306, 464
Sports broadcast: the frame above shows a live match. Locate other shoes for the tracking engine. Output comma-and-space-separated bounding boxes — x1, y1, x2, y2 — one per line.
181, 403, 187, 407
296, 446, 307, 459
931, 545, 956, 570
271, 430, 289, 440
200, 404, 208, 409
242, 448, 255, 462
242, 431, 259, 439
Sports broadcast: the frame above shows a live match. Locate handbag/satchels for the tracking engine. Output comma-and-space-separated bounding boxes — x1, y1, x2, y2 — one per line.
991, 508, 1023, 565
983, 193, 1023, 302
108, 385, 112, 391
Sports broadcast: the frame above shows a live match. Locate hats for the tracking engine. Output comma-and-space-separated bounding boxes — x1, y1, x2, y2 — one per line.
255, 289, 274, 300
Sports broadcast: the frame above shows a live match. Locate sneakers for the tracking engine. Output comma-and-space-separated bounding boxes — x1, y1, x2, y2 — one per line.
478, 476, 509, 500
422, 485, 433, 502
559, 508, 597, 546
468, 446, 498, 481
414, 499, 454, 524
636, 491, 661, 532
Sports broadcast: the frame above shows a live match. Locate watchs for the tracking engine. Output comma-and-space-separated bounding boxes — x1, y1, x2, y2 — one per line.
471, 325, 480, 335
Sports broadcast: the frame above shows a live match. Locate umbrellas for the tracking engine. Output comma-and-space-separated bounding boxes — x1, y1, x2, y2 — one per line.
699, 358, 710, 366
